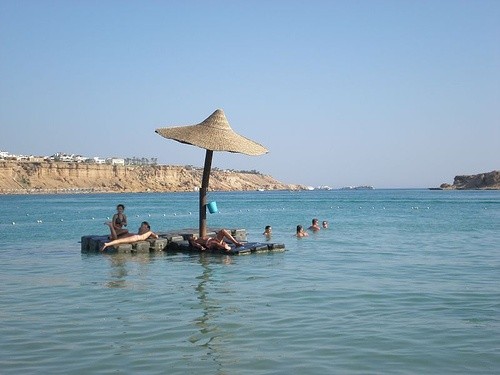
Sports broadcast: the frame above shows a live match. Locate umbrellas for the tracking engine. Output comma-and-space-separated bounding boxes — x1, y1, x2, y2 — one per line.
154, 108, 270, 238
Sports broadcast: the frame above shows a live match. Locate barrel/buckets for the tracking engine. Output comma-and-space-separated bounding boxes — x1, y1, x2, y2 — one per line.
206, 201, 218, 213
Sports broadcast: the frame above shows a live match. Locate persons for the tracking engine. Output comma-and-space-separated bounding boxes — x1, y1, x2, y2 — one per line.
322, 221, 328, 228
188, 228, 244, 251
108, 204, 133, 240
99, 221, 159, 252
296, 225, 309, 237
307, 218, 321, 230
262, 225, 272, 235
